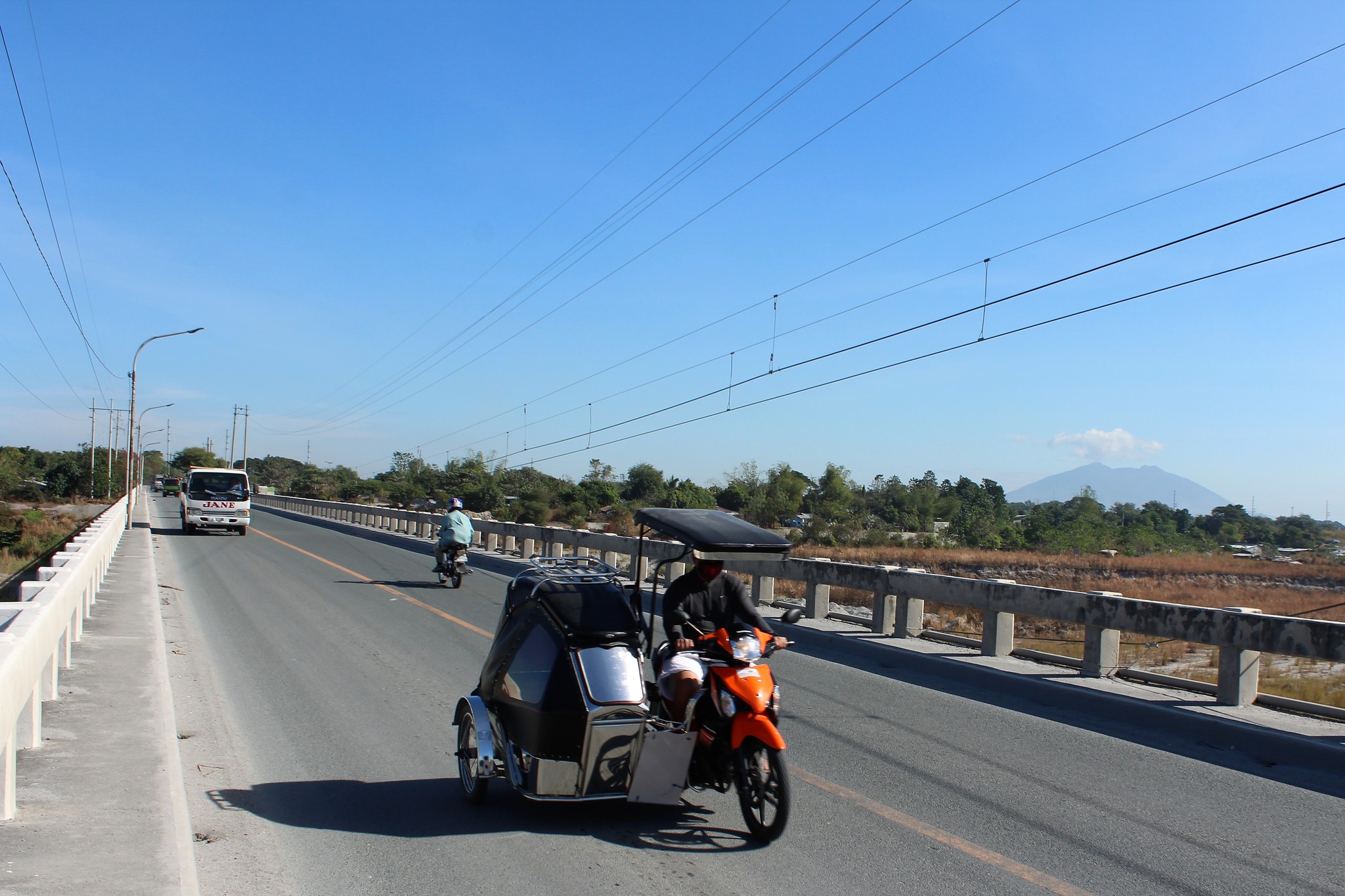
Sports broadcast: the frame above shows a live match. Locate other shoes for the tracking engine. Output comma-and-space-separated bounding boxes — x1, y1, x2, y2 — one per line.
432, 564, 443, 572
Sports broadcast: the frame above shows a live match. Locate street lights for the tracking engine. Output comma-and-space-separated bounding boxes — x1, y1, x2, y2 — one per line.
138, 428, 165, 497
135, 403, 175, 505
126, 327, 206, 533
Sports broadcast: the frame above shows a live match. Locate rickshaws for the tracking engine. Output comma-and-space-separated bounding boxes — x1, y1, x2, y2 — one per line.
451, 509, 803, 842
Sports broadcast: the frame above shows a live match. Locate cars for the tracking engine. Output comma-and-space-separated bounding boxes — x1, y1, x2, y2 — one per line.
151, 481, 154, 491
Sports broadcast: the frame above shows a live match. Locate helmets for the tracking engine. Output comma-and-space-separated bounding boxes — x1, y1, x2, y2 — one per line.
447, 498, 464, 512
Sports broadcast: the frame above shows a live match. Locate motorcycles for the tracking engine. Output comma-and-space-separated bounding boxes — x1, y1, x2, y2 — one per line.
427, 517, 473, 588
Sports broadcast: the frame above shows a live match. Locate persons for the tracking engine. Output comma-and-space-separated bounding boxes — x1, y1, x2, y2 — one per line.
656, 550, 789, 727
163, 475, 179, 480
225, 476, 242, 492
432, 498, 474, 573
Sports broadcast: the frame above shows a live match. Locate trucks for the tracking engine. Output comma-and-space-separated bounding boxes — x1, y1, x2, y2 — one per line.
178, 466, 260, 536
162, 478, 181, 498
153, 474, 168, 493
